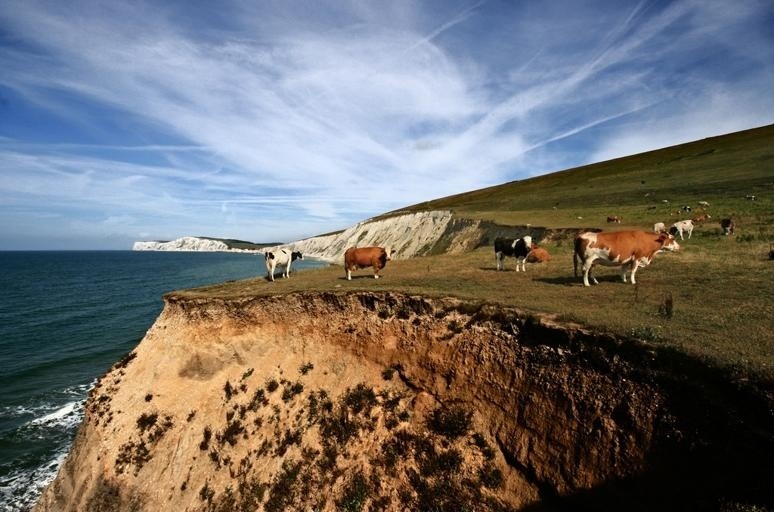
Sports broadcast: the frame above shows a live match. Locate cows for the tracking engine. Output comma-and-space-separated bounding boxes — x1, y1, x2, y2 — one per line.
572, 229, 681, 288
343, 244, 398, 281
606, 216, 624, 225
718, 219, 736, 236
527, 243, 552, 265
264, 248, 304, 282
644, 193, 713, 241
744, 195, 758, 201
494, 234, 533, 273
